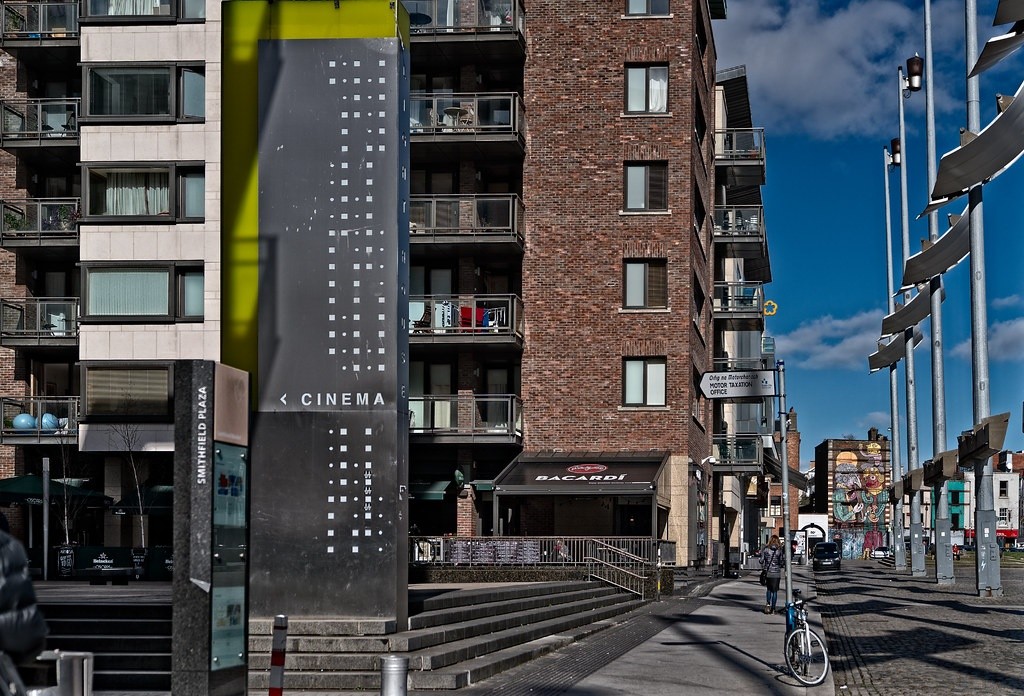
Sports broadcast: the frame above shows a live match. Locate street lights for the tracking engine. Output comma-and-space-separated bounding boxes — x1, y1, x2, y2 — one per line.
868, 138, 924, 574
880, 51, 947, 577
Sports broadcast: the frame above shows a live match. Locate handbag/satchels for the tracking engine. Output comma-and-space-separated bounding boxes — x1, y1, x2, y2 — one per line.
760, 570, 768, 586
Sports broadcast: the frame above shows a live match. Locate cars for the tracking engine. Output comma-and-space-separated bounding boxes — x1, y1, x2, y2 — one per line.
874, 547, 889, 558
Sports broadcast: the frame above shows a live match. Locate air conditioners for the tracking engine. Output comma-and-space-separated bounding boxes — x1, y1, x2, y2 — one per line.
415, 538, 444, 562
432, 302, 459, 333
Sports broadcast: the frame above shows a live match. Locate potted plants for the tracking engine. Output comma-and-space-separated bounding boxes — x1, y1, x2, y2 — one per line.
59, 205, 72, 231
4, 212, 24, 236
9, 11, 23, 38
494, 4, 510, 31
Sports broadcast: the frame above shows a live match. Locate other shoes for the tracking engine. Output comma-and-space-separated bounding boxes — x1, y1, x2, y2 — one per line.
766, 605, 771, 613
771, 609, 776, 615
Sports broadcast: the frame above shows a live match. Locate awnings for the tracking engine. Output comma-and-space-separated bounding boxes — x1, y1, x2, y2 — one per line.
714, 64, 772, 285
489, 447, 672, 511
409, 448, 524, 504
763, 453, 808, 493
708, 0, 728, 21
107, 473, 174, 515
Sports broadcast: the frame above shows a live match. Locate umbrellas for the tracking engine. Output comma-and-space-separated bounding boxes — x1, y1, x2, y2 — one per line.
0, 473, 114, 547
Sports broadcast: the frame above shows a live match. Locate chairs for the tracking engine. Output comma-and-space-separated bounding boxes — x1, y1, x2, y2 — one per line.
461, 109, 475, 132
41, 314, 58, 336
429, 112, 445, 132
32, 112, 77, 136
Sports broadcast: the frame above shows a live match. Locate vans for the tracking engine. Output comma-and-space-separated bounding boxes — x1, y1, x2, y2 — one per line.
813, 542, 842, 571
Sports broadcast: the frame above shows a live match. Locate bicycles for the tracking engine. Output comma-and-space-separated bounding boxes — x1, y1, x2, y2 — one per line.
776, 589, 830, 687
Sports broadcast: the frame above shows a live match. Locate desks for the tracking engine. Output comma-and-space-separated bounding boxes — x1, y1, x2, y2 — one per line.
409, 12, 432, 34
444, 107, 469, 132
85, 567, 133, 585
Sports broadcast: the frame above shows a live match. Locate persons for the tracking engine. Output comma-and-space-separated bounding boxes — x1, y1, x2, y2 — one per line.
922, 540, 936, 561
953, 543, 960, 560
0, 530, 49, 696
754, 535, 799, 616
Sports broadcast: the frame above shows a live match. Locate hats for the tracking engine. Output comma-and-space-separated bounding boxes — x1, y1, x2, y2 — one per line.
865, 443, 882, 450
791, 540, 797, 545
